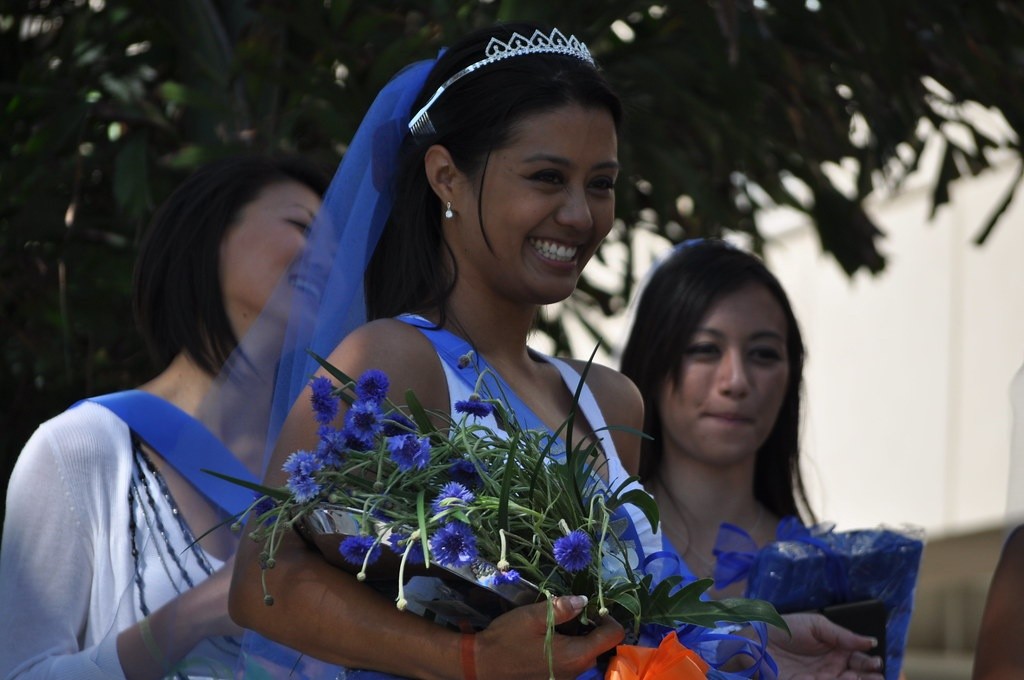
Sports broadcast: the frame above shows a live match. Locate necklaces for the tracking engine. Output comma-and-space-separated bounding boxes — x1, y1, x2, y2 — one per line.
661, 507, 768, 591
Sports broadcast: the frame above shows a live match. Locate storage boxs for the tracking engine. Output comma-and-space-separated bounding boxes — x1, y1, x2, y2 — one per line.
745, 527, 922, 680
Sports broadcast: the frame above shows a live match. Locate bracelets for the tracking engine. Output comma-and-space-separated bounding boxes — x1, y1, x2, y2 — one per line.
462, 633, 477, 680
137, 614, 183, 672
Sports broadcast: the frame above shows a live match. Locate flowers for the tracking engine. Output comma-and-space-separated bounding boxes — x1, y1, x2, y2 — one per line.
184, 340, 792, 680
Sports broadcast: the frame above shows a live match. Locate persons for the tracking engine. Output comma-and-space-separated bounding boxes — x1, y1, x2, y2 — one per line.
972, 522, 1024, 680
0, 150, 347, 680
228, 23, 885, 680
620, 237, 821, 599
